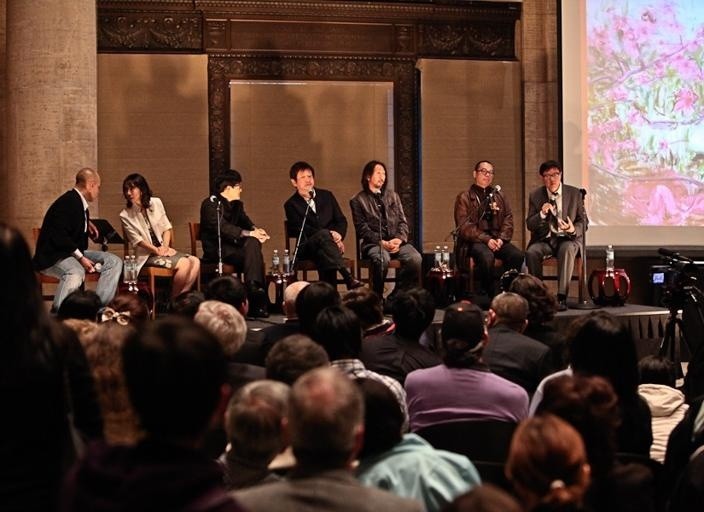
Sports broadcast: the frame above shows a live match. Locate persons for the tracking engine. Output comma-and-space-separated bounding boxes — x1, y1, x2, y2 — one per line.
200, 168, 271, 318
525, 159, 589, 311
118, 172, 201, 298
453, 159, 524, 298
32, 167, 123, 317
1, 223, 704, 512
282, 160, 365, 291
349, 159, 423, 295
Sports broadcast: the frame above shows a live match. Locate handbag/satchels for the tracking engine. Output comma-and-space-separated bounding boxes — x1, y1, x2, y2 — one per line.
454, 237, 472, 280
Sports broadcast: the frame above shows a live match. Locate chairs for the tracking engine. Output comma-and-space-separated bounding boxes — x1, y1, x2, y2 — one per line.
284, 219, 355, 290
356, 229, 400, 283
123, 220, 175, 319
189, 223, 244, 292
531, 189, 587, 303
453, 231, 503, 292
33, 227, 99, 301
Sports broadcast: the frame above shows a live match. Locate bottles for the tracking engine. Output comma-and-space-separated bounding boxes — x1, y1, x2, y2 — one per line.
605, 244, 615, 272
123, 255, 131, 284
434, 245, 442, 271
283, 249, 291, 276
130, 255, 139, 286
271, 249, 280, 276
442, 245, 450, 270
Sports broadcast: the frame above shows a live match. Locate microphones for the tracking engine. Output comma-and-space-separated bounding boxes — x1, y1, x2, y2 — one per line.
374, 189, 385, 211
547, 194, 555, 219
307, 187, 316, 201
487, 185, 501, 199
210, 196, 223, 207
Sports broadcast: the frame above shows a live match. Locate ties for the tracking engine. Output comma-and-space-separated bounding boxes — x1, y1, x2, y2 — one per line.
549, 192, 560, 251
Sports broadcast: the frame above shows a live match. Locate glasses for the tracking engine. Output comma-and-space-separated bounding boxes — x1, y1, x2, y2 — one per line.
476, 168, 495, 177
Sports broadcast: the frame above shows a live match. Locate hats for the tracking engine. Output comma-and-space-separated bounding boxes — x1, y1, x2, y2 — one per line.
440, 297, 489, 355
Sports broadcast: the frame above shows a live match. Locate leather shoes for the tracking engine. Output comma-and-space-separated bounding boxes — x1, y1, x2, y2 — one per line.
248, 306, 270, 319
345, 274, 365, 291
556, 298, 569, 313
243, 281, 266, 296
